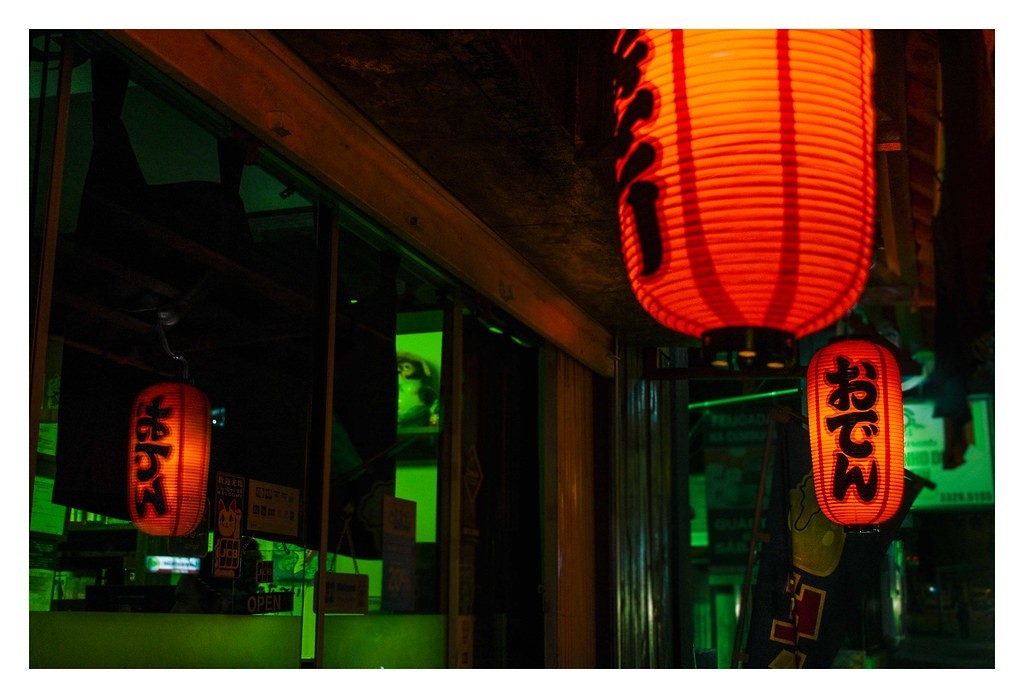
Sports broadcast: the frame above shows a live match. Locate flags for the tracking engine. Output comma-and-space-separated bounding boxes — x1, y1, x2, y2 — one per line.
742, 421, 922, 669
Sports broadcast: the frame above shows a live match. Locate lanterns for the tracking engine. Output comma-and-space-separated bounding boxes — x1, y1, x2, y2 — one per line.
610, 30, 876, 366
129, 375, 212, 543
806, 336, 904, 535
